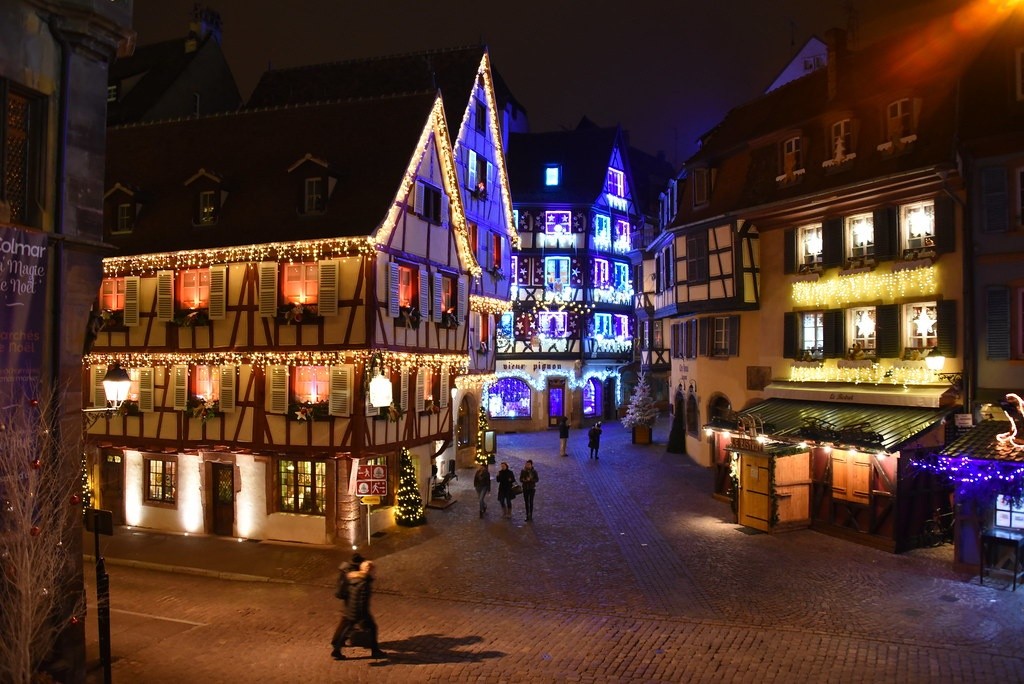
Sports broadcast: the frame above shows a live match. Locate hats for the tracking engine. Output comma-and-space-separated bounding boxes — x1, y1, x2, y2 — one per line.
596, 421, 601, 428
352, 553, 365, 563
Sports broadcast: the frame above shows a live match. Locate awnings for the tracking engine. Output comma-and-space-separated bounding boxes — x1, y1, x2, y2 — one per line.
937, 419, 1024, 464
701, 397, 964, 455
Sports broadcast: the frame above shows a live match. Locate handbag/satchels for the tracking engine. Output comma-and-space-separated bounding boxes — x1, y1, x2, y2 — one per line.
512, 483, 522, 495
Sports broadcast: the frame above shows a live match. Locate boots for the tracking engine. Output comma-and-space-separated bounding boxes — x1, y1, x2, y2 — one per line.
501, 507, 506, 517
507, 508, 512, 518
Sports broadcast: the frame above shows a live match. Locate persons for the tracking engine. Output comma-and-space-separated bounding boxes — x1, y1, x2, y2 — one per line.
519, 460, 540, 521
330, 561, 390, 660
558, 417, 571, 457
473, 463, 491, 519
496, 462, 516, 519
588, 421, 603, 459
331, 553, 380, 648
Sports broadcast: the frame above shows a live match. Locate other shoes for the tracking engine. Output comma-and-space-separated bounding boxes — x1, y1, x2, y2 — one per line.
590, 456, 593, 459
483, 506, 487, 513
530, 515, 532, 520
331, 651, 346, 659
524, 516, 529, 522
480, 512, 483, 519
372, 651, 387, 658
595, 457, 599, 460
561, 454, 568, 457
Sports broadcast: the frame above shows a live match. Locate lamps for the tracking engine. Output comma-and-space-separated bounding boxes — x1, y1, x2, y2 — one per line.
84, 363, 132, 416
366, 362, 390, 408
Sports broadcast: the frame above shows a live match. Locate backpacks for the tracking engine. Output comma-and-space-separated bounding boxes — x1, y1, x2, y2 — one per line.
335, 572, 350, 599
